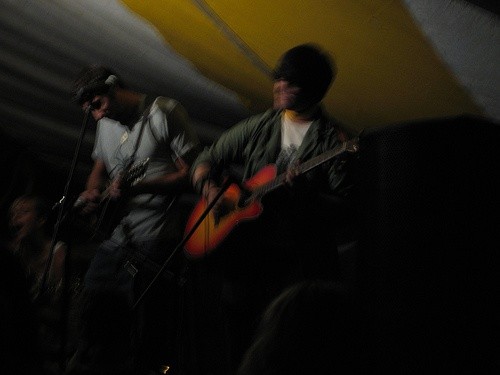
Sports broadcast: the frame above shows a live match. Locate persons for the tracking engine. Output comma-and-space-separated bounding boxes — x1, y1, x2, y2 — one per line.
0, 192, 83, 375
191, 45, 357, 375
236, 279, 338, 375
64, 64, 204, 375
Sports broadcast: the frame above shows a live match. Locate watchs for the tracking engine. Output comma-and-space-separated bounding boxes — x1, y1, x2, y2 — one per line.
194, 174, 214, 195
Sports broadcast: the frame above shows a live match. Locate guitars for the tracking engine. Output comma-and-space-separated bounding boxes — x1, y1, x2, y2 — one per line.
182, 135, 360, 260
61, 157, 150, 246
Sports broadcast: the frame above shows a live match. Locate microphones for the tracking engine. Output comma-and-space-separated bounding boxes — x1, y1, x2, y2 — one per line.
87, 74, 118, 108
